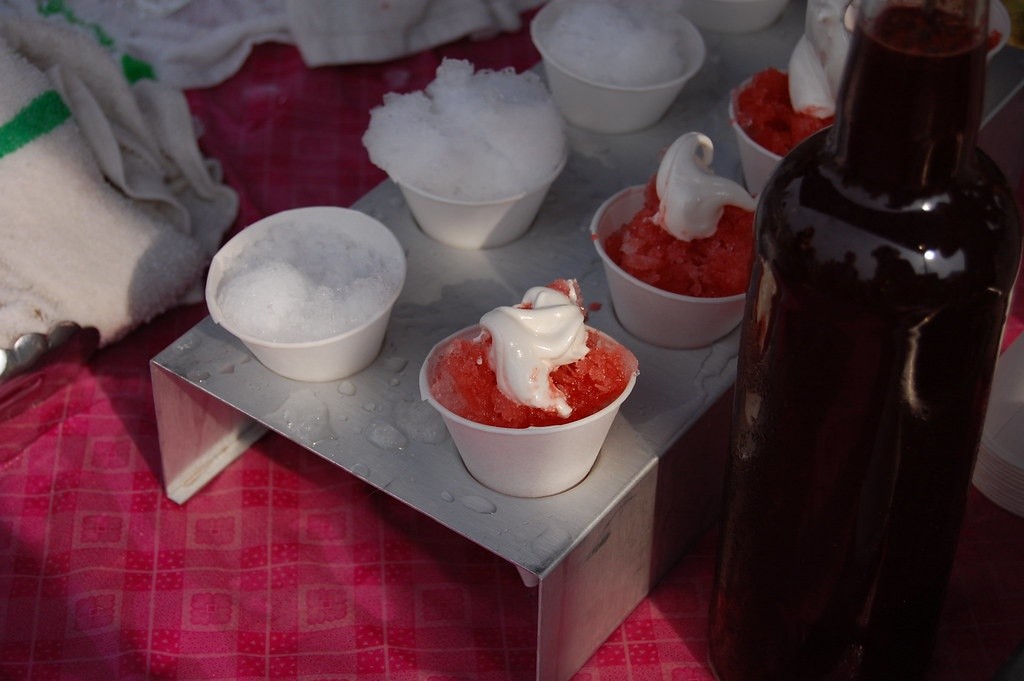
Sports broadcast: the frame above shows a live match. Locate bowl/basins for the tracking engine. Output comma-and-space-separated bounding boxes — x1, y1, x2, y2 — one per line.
590, 185, 757, 350
381, 142, 567, 251
985, 0, 1011, 66
420, 319, 638, 498
680, 0, 789, 32
729, 69, 787, 197
204, 207, 407, 383
529, 0, 706, 135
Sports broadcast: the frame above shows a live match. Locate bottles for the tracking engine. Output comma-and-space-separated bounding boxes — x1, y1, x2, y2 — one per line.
707, 0, 1022, 680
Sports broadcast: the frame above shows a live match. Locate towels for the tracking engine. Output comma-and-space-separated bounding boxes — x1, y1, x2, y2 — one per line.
0, 0, 547, 354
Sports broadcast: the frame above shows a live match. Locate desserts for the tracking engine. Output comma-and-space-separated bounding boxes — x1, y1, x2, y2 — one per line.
788, 0, 851, 118
650, 131, 760, 242
478, 287, 589, 417
